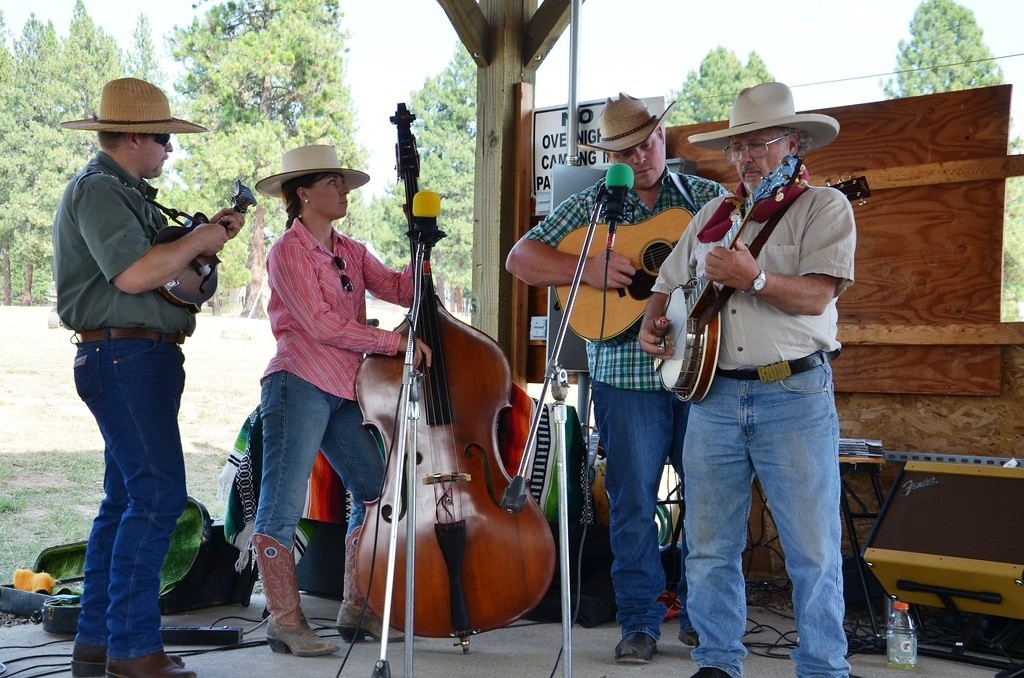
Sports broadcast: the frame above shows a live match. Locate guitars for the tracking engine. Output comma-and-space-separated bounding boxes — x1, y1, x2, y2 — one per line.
149, 179, 258, 315
551, 172, 874, 348
652, 152, 805, 405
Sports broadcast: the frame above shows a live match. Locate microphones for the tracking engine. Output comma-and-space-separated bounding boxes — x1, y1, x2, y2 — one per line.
412, 191, 441, 249
605, 164, 635, 260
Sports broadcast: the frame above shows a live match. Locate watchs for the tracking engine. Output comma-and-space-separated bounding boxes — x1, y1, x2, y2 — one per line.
744, 268, 766, 296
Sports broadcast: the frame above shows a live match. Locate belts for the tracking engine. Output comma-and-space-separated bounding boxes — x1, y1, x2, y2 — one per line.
78, 329, 186, 345
715, 349, 841, 383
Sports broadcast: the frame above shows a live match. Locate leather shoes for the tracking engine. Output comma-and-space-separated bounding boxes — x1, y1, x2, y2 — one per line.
70, 642, 185, 677
615, 630, 657, 663
678, 629, 699, 648
105, 650, 197, 678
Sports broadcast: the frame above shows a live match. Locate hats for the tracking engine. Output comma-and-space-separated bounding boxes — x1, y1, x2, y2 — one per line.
58, 78, 210, 134
256, 145, 369, 198
686, 83, 841, 152
575, 91, 677, 155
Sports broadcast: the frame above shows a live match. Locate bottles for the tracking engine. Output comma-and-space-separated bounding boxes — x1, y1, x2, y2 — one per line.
886, 602, 920, 671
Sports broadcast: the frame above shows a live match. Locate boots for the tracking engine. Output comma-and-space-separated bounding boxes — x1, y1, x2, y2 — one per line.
335, 526, 405, 643
249, 531, 340, 657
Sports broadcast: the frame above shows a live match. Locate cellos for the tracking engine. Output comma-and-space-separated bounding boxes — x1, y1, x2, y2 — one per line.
353, 98, 560, 654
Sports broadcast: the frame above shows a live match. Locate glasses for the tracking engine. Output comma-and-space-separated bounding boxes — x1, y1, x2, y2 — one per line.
333, 255, 355, 292
723, 131, 794, 162
152, 133, 171, 146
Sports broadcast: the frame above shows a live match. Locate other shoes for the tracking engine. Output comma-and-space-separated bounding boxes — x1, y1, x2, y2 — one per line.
691, 666, 732, 678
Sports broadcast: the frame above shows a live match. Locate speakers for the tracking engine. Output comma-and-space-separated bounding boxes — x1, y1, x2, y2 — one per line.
859, 459, 1024, 619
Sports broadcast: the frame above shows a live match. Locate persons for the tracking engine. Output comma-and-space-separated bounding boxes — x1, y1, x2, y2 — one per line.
505, 92, 732, 663
639, 82, 856, 678
55, 78, 245, 678
252, 145, 423, 658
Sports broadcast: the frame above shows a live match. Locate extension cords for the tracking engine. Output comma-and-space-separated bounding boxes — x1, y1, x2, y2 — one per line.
157, 626, 243, 644
